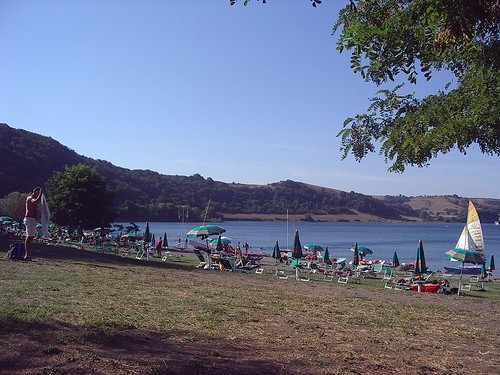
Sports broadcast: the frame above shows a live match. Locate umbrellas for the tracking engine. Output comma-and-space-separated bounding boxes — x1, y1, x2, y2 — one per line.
0, 216, 55, 231
271, 240, 281, 263
442, 248, 488, 296
292, 229, 330, 267
490, 253, 495, 272
185, 224, 226, 247
349, 242, 374, 267
162, 232, 169, 247
413, 239, 428, 281
94, 222, 155, 244
392, 251, 399, 268
209, 235, 232, 252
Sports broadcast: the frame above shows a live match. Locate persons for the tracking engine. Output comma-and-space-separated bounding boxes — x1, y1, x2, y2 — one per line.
237, 242, 241, 252
95, 235, 120, 247
23, 187, 43, 261
154, 237, 163, 258
243, 242, 249, 253
395, 276, 448, 285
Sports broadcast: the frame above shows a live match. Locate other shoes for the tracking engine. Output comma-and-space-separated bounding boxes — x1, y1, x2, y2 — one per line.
24, 257, 31, 261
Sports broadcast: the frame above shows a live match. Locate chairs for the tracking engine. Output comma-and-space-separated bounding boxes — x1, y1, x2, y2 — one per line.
67, 237, 493, 295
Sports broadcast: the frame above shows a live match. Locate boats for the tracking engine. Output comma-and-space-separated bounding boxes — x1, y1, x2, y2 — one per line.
410, 283, 440, 293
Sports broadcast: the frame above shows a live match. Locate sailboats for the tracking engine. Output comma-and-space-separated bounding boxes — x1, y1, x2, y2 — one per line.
444, 200, 488, 274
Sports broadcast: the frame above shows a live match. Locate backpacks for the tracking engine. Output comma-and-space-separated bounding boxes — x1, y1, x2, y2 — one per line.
8, 242, 23, 259
